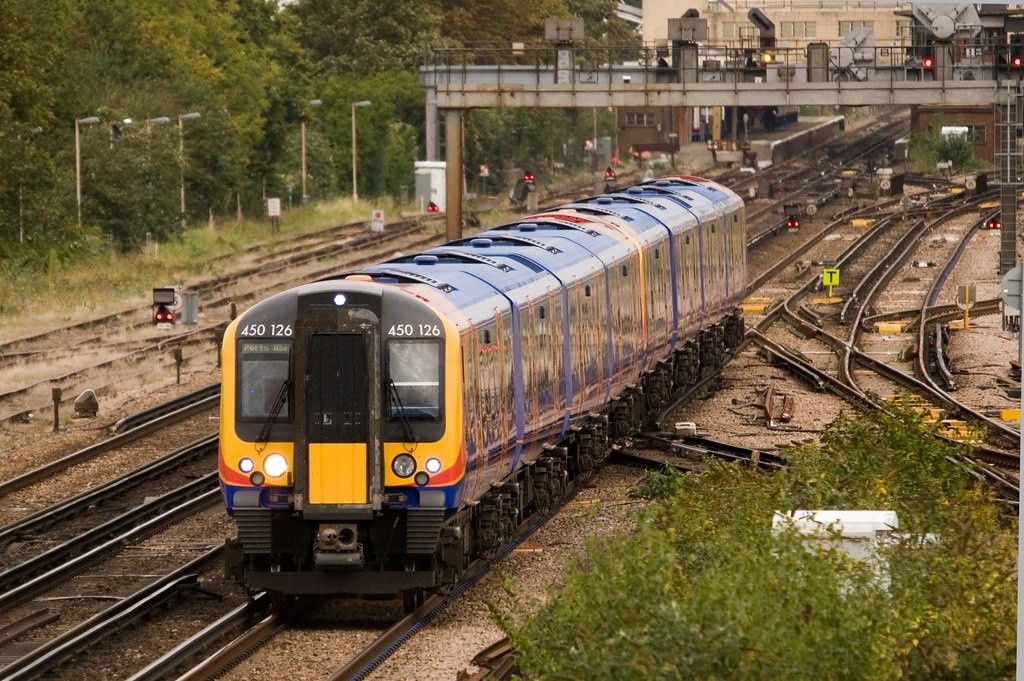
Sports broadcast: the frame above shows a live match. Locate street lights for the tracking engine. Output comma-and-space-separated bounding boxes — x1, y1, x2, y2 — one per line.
180, 111, 201, 232
76, 116, 99, 228
141, 115, 168, 166
300, 98, 323, 207
107, 116, 132, 151
353, 98, 373, 207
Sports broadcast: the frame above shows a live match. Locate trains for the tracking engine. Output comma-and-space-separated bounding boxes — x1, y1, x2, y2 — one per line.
217, 171, 745, 627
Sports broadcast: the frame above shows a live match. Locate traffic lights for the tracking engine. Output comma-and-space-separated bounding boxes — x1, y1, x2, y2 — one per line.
523, 174, 535, 180
924, 57, 934, 68
604, 171, 615, 176
786, 221, 799, 227
761, 52, 774, 63
988, 221, 1001, 229
1012, 57, 1022, 67
427, 205, 439, 211
